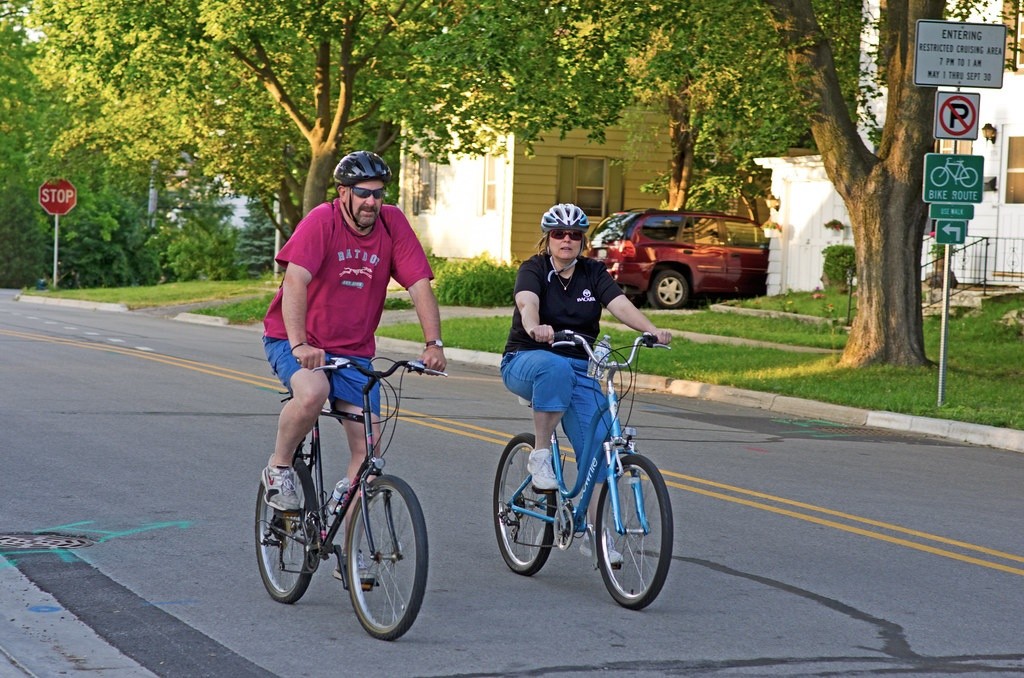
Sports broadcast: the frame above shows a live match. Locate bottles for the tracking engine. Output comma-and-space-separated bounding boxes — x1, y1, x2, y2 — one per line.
586, 334, 610, 380
328, 478, 350, 513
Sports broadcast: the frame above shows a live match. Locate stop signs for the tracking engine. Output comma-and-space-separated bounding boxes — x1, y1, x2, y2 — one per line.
38, 178, 77, 215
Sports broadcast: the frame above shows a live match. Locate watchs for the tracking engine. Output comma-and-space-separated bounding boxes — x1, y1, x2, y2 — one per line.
426, 339, 444, 348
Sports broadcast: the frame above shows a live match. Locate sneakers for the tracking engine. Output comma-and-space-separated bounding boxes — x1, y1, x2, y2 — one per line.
527, 448, 560, 489
579, 524, 624, 564
261, 454, 302, 512
332, 478, 351, 502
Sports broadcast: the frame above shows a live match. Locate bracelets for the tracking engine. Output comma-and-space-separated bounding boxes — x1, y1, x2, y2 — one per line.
291, 342, 308, 355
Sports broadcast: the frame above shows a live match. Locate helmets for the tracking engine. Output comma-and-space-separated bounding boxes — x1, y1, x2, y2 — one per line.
333, 150, 393, 188
541, 203, 590, 232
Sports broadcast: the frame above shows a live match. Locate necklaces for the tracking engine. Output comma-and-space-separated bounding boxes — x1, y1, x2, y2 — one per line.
551, 256, 577, 290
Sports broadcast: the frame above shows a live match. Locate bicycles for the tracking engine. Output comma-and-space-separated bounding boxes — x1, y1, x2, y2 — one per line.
492, 329, 675, 610
253, 355, 448, 642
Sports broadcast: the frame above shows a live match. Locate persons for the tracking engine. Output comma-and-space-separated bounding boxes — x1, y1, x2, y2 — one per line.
261, 150, 448, 581
500, 203, 672, 565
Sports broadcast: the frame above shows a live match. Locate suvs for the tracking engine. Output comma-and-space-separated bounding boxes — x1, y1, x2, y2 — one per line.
588, 208, 770, 310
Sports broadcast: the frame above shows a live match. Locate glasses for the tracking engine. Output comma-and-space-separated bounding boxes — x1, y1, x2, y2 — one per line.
551, 229, 583, 240
351, 186, 386, 199
